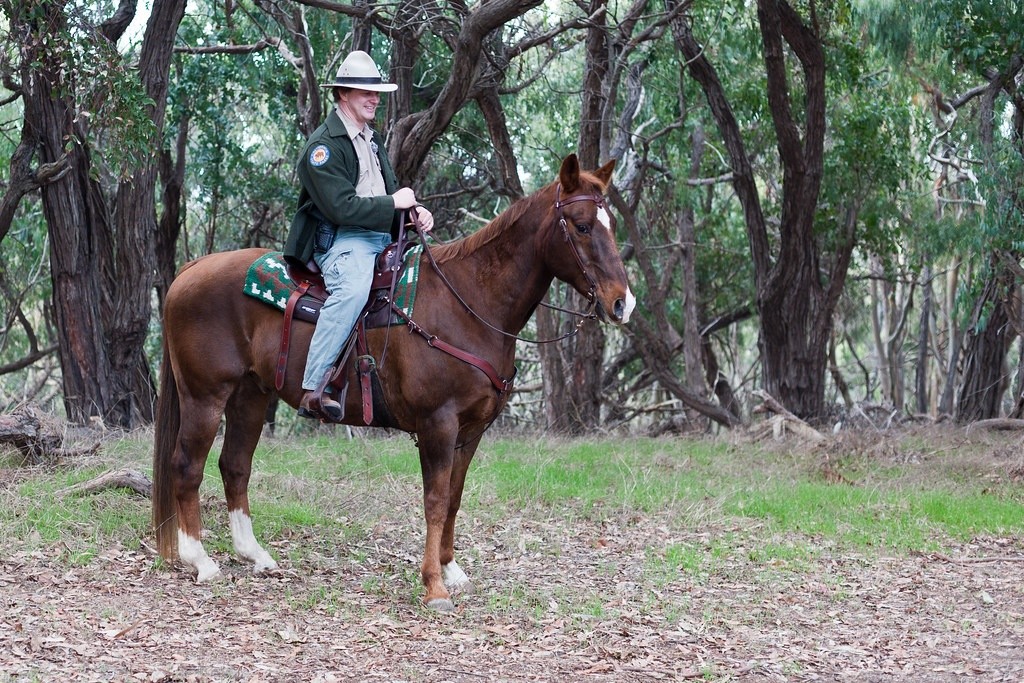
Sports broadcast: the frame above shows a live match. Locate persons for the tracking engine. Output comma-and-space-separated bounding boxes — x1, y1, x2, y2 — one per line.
283, 49, 434, 420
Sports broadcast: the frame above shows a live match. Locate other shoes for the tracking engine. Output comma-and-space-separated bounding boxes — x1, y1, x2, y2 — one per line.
297, 390, 341, 418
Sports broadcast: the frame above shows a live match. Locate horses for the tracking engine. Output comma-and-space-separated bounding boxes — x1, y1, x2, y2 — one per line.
151, 154, 636, 614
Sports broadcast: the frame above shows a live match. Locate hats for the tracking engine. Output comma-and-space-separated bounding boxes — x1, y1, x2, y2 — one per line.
319, 50, 398, 92
